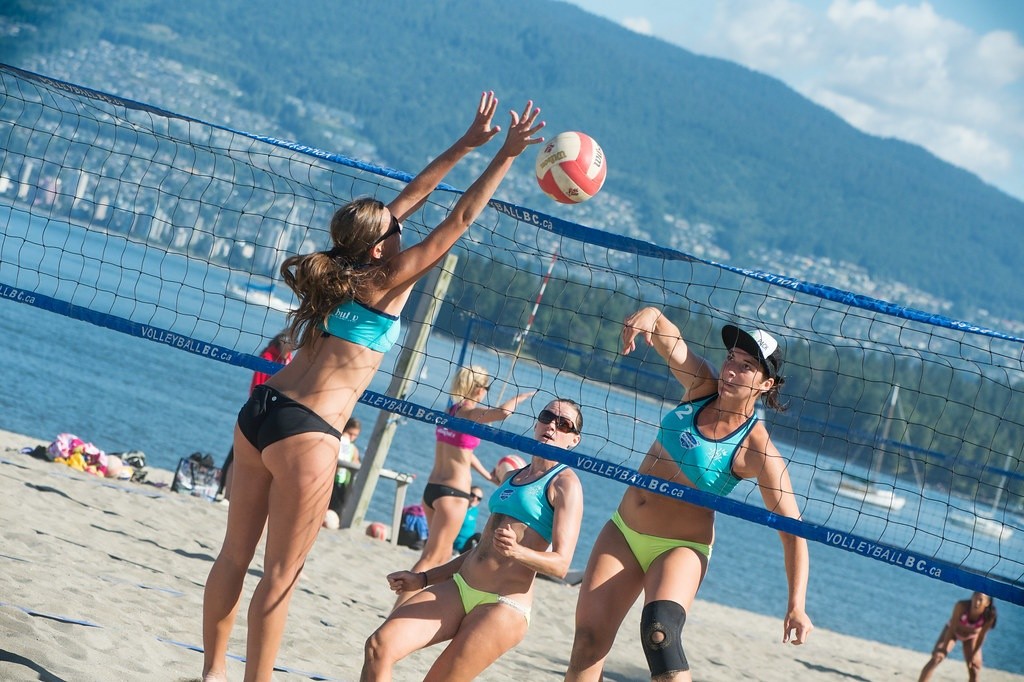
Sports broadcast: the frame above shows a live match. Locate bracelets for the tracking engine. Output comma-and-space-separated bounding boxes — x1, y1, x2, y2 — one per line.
423, 572, 427, 587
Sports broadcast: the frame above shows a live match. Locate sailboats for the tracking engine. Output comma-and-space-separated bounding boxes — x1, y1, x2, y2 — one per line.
943, 447, 1016, 540
820, 380, 924, 513
218, 206, 300, 315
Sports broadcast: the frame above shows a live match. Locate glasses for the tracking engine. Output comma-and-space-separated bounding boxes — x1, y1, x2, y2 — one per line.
538, 410, 579, 434
470, 492, 482, 502
369, 216, 402, 246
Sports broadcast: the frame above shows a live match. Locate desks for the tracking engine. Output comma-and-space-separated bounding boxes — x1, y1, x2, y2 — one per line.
339, 459, 414, 545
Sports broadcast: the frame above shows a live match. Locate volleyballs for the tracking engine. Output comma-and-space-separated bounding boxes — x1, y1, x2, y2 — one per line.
365, 524, 385, 541
536, 131, 607, 203
322, 509, 339, 529
496, 454, 527, 484
105, 455, 122, 479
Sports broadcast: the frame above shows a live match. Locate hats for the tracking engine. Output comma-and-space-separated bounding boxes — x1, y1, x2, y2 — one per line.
721, 320, 783, 379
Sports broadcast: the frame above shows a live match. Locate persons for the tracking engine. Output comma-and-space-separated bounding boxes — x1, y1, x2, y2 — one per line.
559, 306, 814, 682
213, 334, 294, 501
328, 418, 362, 517
919, 591, 996, 682
391, 366, 537, 614
365, 399, 583, 682
202, 90, 546, 682
453, 486, 483, 554
